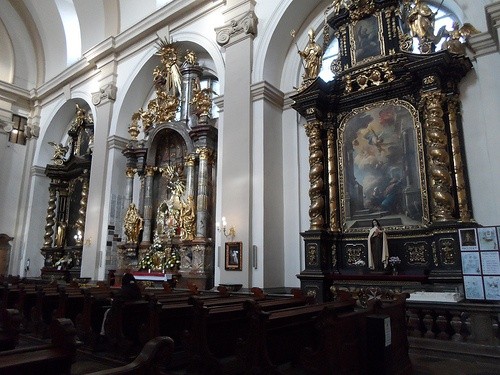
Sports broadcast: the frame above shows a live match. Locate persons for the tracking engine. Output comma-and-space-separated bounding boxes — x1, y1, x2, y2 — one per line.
50, 102, 95, 162
100, 273, 140, 337
297, 28, 322, 80
408, 0, 435, 44
123, 203, 144, 243
179, 194, 196, 241
368, 219, 389, 272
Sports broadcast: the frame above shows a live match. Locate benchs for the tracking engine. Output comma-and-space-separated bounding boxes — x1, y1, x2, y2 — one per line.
0, 272, 411, 375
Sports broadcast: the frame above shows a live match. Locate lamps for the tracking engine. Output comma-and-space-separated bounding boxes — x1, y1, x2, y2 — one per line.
74, 230, 83, 246
220, 216, 236, 239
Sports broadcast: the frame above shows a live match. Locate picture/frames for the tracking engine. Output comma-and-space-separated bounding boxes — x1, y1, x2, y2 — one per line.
223, 241, 243, 272
